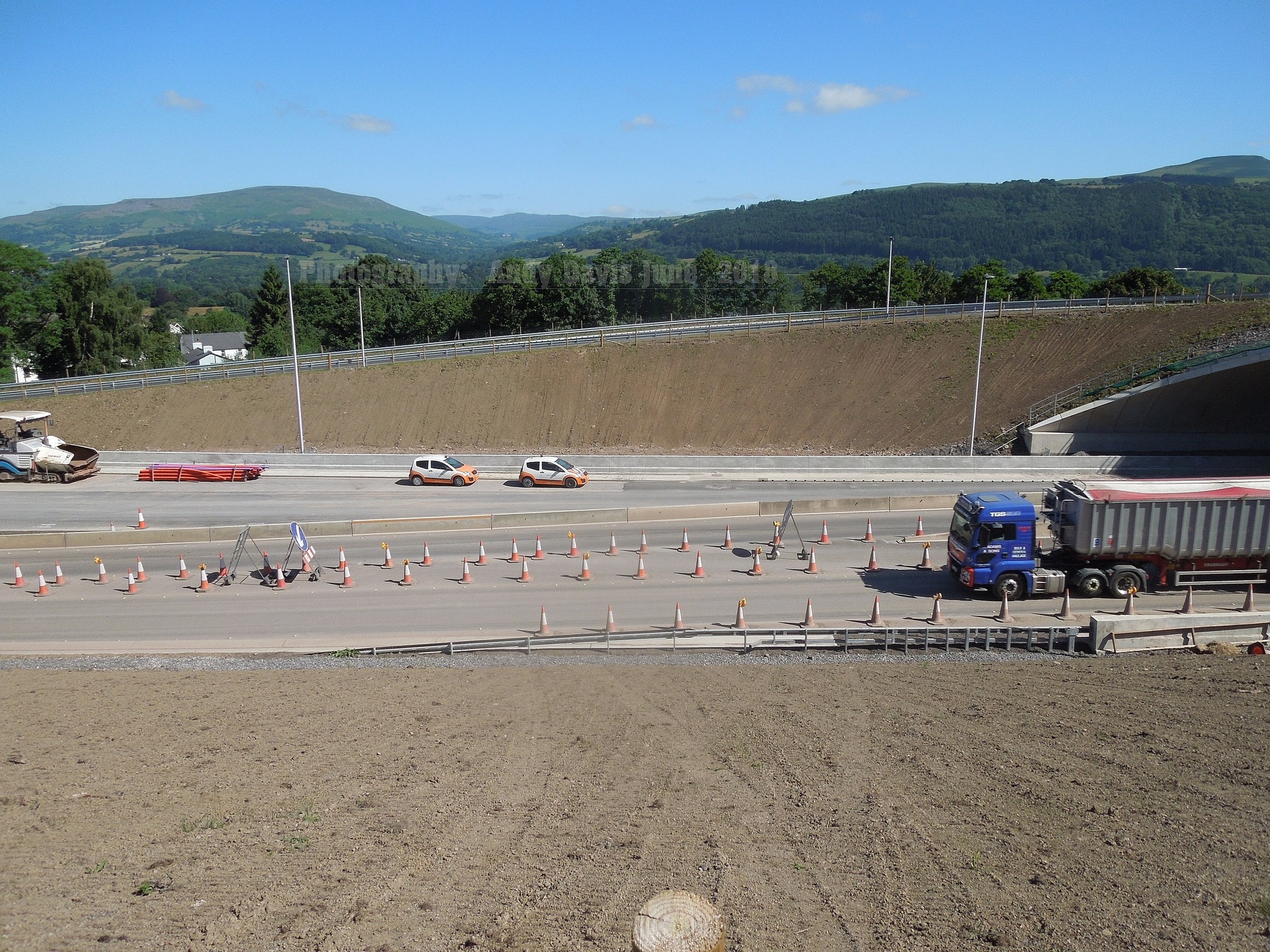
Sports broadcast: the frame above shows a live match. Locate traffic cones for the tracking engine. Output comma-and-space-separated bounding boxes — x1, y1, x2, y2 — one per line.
865, 594, 888, 628
600, 605, 620, 633
731, 600, 750, 630
1116, 592, 1139, 615
1174, 586, 1199, 614
534, 606, 546, 639
1053, 588, 1077, 620
799, 597, 819, 629
926, 592, 947, 625
669, 602, 688, 632
993, 593, 1015, 623
1236, 583, 1260, 612
863, 545, 882, 573
9, 515, 942, 597
916, 547, 935, 570
134, 507, 150, 530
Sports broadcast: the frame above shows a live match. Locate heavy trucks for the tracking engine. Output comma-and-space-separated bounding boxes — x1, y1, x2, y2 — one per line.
945, 475, 1270, 602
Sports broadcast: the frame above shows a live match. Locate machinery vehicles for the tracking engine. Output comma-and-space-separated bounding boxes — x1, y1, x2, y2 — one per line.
0, 407, 101, 484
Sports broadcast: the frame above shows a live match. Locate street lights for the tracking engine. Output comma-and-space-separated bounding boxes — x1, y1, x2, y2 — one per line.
357, 283, 366, 367
967, 273, 995, 458
885, 235, 895, 316
284, 253, 305, 454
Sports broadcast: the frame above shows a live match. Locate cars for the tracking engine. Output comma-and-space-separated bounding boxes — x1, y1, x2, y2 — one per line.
409, 455, 479, 487
518, 454, 589, 489
